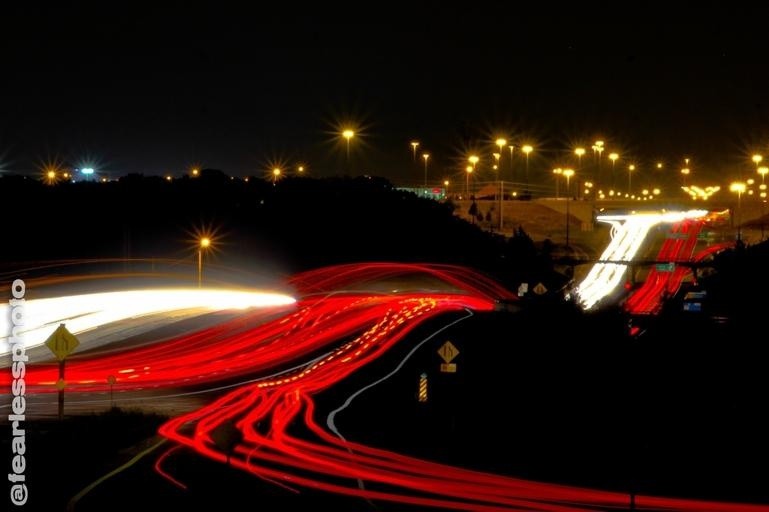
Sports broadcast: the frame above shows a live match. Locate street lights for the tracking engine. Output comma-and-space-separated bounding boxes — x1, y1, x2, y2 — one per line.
341, 129, 353, 176
197, 237, 211, 286
273, 168, 281, 183
81, 168, 94, 182
